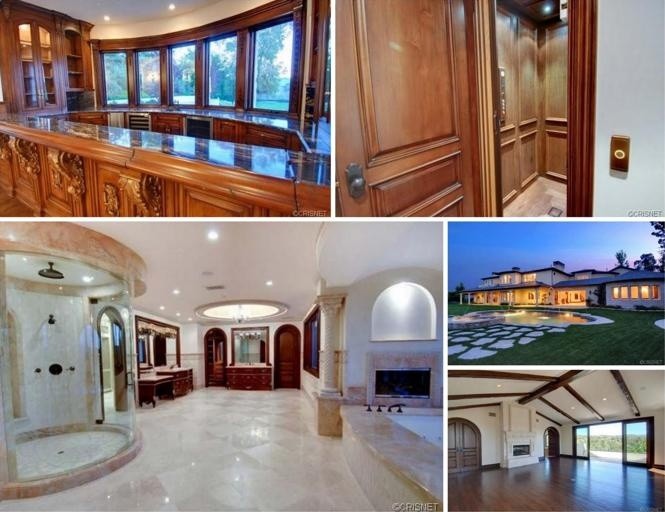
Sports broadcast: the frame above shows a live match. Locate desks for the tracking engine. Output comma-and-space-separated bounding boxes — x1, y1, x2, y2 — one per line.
138, 375, 176, 408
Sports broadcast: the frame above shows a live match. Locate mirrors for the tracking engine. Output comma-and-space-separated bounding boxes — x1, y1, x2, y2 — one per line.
230, 326, 271, 367
135, 315, 181, 376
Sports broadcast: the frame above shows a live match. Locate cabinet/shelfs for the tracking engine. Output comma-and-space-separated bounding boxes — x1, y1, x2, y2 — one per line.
0, 0, 63, 116
69, 113, 107, 127
52, 11, 92, 91
213, 118, 290, 151
224, 366, 273, 391
157, 367, 195, 399
151, 113, 186, 135
